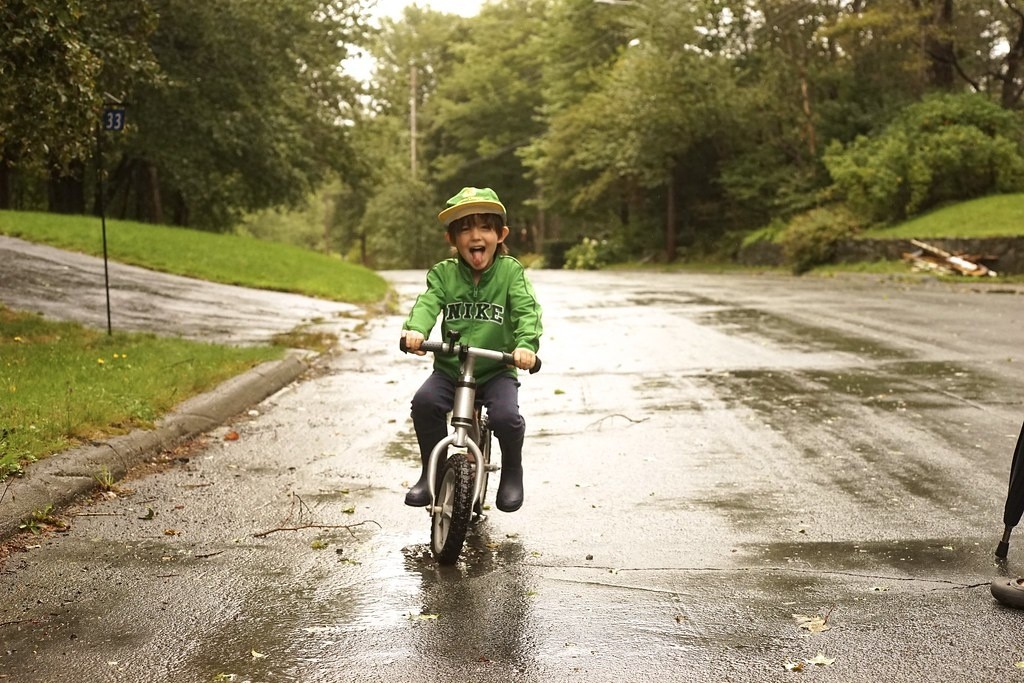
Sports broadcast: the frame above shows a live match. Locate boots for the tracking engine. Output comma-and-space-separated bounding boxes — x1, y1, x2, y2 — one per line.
496, 435, 524, 512
405, 435, 448, 506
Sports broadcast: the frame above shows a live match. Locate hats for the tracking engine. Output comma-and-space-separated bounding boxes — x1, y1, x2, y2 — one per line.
438, 187, 507, 226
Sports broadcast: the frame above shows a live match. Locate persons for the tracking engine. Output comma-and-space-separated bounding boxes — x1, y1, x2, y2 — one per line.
400, 186, 541, 512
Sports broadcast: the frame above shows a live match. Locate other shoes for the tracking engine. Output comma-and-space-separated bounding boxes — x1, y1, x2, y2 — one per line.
991, 577, 1024, 606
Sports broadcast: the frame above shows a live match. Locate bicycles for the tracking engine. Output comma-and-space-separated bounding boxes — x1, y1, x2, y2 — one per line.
400, 330, 542, 566
